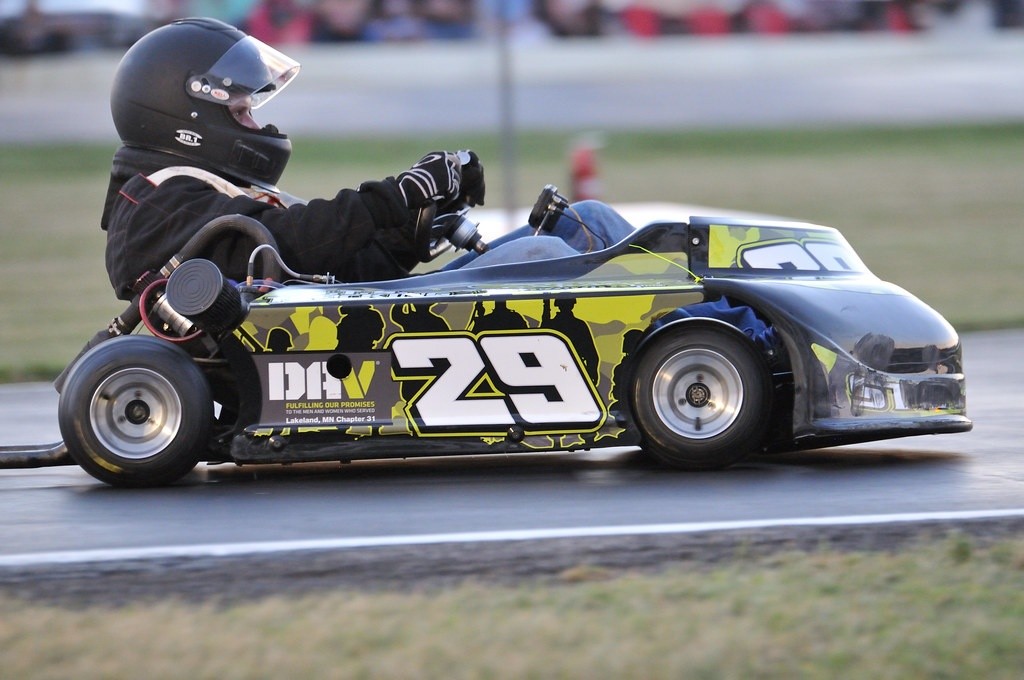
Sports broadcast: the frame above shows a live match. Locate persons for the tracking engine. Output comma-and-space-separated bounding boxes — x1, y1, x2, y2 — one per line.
101, 17, 636, 300
0, 0, 1024, 59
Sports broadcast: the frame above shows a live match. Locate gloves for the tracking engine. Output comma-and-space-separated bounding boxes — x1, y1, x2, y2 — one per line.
394, 151, 462, 214
427, 152, 485, 216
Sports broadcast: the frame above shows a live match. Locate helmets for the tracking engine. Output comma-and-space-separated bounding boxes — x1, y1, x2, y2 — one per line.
109, 17, 292, 195
